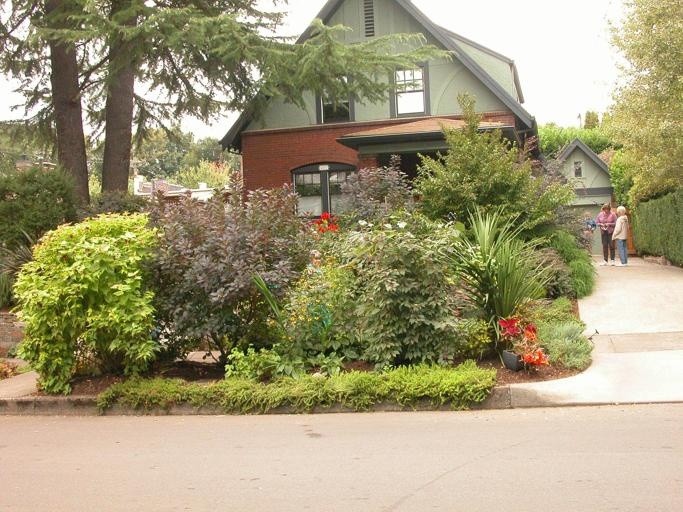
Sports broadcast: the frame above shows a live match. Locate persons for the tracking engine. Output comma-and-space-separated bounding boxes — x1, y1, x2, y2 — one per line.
578, 212, 597, 258
594, 204, 618, 266
611, 205, 629, 267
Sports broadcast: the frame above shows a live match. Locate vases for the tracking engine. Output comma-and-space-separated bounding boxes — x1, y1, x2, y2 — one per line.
502, 350, 525, 372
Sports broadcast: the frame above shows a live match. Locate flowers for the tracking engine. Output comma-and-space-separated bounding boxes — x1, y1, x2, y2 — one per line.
500, 317, 550, 367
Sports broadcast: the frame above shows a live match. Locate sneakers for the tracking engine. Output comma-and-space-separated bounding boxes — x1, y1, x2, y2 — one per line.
618, 264, 627, 266
611, 260, 615, 265
600, 261, 607, 266
